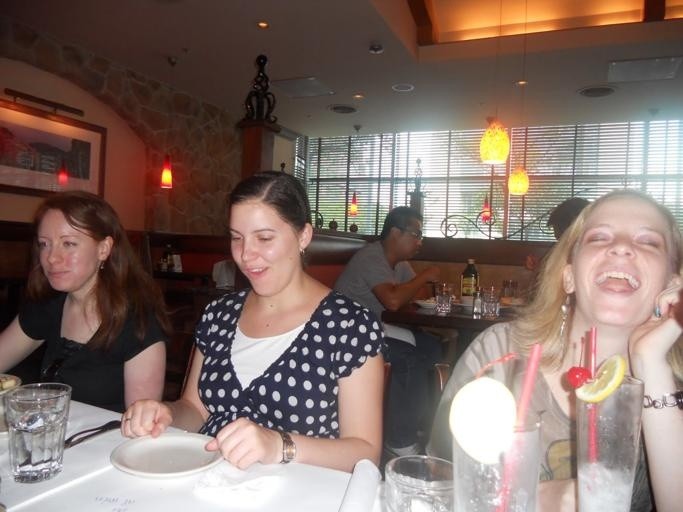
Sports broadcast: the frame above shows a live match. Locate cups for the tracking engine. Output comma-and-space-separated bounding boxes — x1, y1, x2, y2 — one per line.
4, 382, 73, 484
574, 374, 644, 512
449, 408, 541, 512
383, 452, 453, 512
439, 282, 448, 295
435, 295, 450, 313
0, 374, 22, 434
482, 286, 499, 317
502, 279, 518, 296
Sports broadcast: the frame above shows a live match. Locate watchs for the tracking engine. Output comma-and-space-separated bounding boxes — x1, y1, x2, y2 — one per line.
278, 429, 296, 465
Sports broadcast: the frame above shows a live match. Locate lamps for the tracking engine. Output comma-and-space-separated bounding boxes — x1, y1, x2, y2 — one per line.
507, 0, 532, 196
478, 1, 510, 164
348, 124, 359, 216
160, 56, 174, 190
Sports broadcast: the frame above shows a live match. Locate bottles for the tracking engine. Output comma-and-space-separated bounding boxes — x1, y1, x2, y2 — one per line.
472, 286, 484, 314
164, 244, 171, 271
462, 258, 477, 296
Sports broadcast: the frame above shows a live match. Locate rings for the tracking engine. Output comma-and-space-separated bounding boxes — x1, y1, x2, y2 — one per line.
125, 419, 130, 421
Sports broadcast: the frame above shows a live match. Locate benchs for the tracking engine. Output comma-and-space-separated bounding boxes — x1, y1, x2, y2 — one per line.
139, 231, 232, 291
305, 234, 368, 290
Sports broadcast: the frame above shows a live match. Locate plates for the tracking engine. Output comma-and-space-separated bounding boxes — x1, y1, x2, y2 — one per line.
414, 299, 435, 309
453, 303, 471, 311
501, 305, 511, 309
108, 433, 226, 478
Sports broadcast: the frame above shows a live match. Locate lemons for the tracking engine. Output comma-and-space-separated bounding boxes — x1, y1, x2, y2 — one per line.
575, 354, 626, 405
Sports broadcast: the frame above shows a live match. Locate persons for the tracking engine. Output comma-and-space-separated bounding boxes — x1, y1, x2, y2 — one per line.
121, 172, 391, 477
1, 188, 167, 411
526, 199, 590, 273
424, 186, 682, 512
330, 204, 444, 460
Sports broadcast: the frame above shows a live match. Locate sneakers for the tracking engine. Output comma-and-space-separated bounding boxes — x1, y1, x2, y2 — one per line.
383, 441, 424, 460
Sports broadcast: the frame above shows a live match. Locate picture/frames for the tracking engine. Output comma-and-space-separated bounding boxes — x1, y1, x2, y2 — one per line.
0, 97, 107, 204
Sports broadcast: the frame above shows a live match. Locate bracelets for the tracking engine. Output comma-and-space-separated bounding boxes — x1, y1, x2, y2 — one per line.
641, 390, 683, 411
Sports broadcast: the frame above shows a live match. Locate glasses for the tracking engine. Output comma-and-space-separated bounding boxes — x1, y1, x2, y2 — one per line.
397, 226, 422, 239
